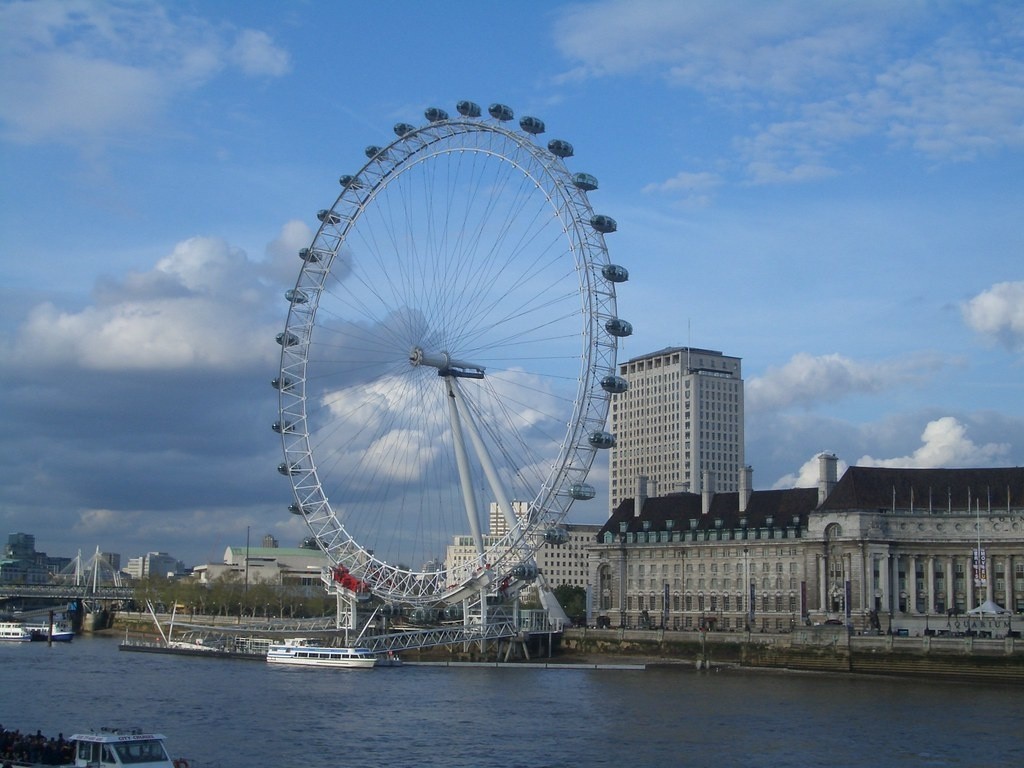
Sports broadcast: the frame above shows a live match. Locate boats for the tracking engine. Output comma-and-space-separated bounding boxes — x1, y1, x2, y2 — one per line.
0, 727, 179, 768
266, 639, 383, 669
375, 649, 403, 668
0, 621, 33, 642
26, 621, 77, 642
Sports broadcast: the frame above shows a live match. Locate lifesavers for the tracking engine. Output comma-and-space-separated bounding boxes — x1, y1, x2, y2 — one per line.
176, 760, 188, 768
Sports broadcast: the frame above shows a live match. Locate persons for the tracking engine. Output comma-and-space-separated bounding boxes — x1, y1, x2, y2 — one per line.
0, 724, 77, 768
